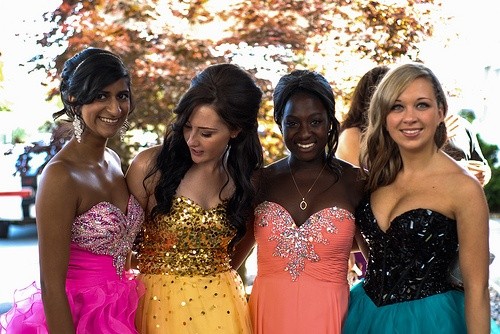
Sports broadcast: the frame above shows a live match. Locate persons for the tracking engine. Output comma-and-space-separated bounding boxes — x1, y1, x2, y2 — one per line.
342, 64, 491, 334
335, 66, 390, 281
230, 69, 372, 334
124, 63, 264, 334
0, 47, 146, 334
444, 113, 492, 188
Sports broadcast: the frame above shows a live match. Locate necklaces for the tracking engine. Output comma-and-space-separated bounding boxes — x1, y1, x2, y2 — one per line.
284, 157, 328, 210
